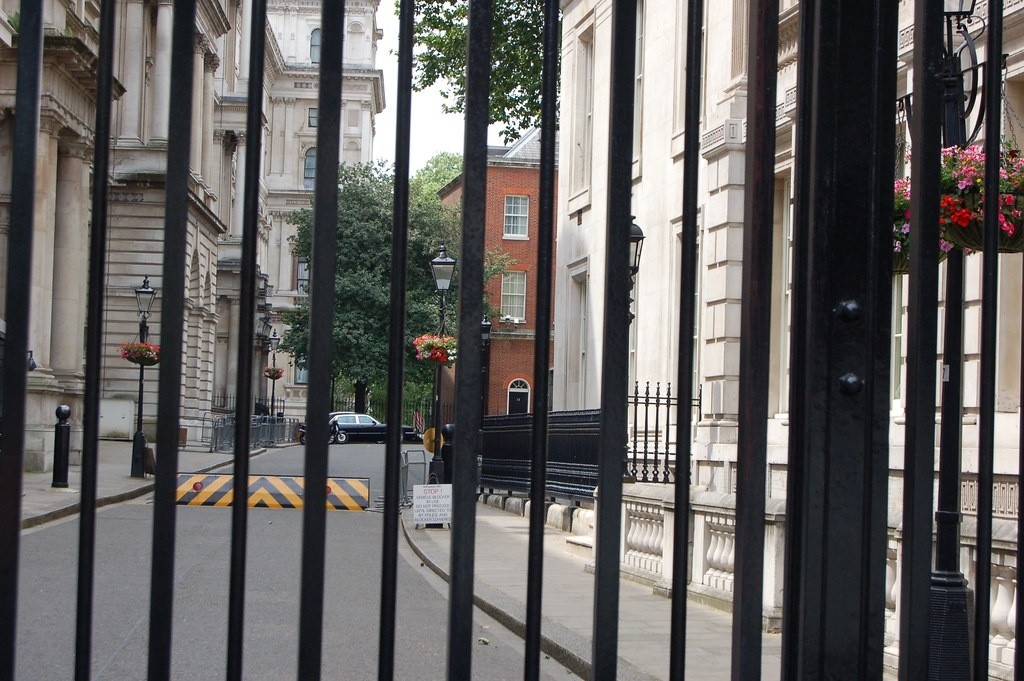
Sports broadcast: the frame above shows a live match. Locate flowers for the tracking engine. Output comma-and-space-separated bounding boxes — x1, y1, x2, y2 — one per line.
895, 136, 1024, 248
265, 368, 283, 377
116, 342, 161, 361
412, 332, 458, 361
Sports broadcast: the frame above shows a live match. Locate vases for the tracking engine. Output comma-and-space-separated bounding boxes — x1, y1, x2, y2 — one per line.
940, 221, 1024, 256
269, 377, 280, 380
892, 243, 947, 273
429, 359, 453, 367
128, 358, 157, 366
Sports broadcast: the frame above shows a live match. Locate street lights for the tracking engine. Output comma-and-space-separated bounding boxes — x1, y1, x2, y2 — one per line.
623, 214, 645, 485
268, 330, 282, 443
130, 273, 159, 477
931, 0, 978, 681
425, 244, 457, 528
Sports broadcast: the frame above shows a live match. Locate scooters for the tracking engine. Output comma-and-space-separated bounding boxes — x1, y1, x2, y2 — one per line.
298, 418, 349, 445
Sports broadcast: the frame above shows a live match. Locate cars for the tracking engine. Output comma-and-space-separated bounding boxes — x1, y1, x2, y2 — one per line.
328, 411, 417, 444
